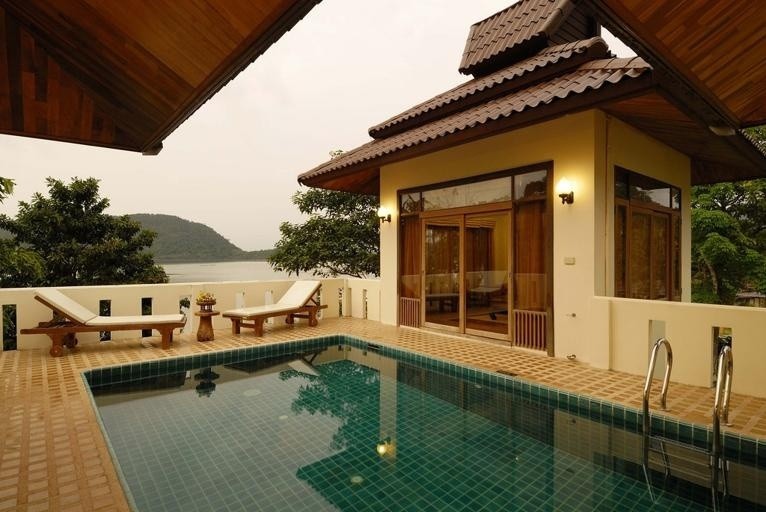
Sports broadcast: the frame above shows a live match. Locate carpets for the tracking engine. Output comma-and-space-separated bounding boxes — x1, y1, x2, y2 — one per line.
448, 308, 508, 334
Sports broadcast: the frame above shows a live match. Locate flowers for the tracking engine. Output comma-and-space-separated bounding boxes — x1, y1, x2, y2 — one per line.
196, 292, 215, 305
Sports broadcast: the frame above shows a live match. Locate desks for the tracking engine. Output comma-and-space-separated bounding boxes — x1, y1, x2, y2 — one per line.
426, 293, 459, 313
470, 287, 501, 306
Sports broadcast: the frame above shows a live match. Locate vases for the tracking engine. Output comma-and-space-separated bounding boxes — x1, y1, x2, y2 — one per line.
201, 305, 212, 313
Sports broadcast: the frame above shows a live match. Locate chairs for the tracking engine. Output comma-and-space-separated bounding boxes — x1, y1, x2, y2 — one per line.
221, 280, 328, 337
20, 289, 188, 357
